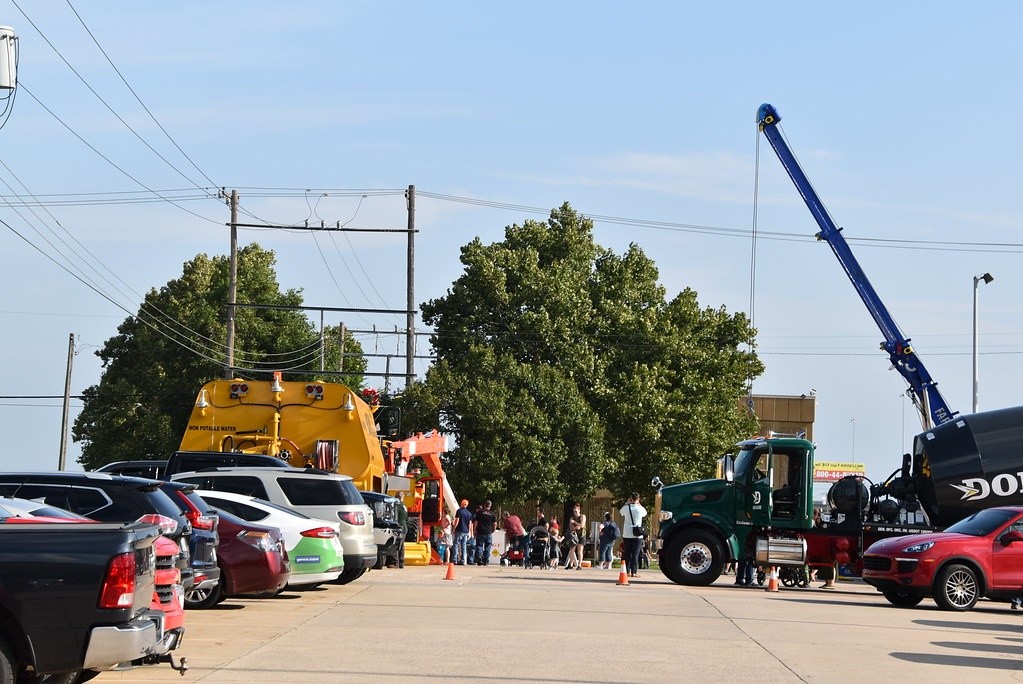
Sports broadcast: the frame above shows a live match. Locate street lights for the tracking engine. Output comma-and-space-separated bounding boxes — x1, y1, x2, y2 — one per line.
899, 393, 905, 454
972, 272, 994, 414
851, 418, 856, 462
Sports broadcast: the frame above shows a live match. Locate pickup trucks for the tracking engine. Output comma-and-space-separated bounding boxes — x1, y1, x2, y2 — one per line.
0, 523, 163, 684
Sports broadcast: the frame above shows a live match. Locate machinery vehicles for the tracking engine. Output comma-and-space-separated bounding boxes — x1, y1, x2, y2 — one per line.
652, 100, 1023, 586
179, 370, 462, 566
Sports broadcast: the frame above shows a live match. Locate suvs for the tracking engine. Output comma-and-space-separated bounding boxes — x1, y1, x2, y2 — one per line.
0, 471, 224, 609
92, 459, 167, 481
862, 506, 1023, 612
169, 467, 378, 586
164, 450, 410, 569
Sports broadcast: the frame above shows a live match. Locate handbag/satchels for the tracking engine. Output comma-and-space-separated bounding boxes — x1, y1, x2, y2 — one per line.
633, 526, 645, 536
599, 524, 621, 545
564, 529, 578, 549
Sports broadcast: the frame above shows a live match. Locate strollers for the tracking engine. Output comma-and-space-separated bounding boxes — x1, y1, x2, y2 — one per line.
524, 525, 553, 571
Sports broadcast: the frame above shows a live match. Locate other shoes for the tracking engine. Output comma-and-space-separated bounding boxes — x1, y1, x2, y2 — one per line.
1011, 605, 1021, 609
631, 573, 639, 577
721, 570, 729, 575
735, 572, 738, 575
565, 566, 574, 569
731, 577, 744, 587
818, 585, 835, 589
627, 572, 630, 576
744, 577, 759, 587
576, 566, 582, 570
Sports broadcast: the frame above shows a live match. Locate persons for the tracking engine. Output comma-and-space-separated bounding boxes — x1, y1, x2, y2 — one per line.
389, 490, 408, 568
436, 499, 654, 570
619, 492, 647, 577
1011, 597, 1023, 609
721, 507, 839, 589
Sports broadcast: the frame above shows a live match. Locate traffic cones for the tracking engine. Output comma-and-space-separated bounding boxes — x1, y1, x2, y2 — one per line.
443, 563, 457, 580
765, 566, 780, 592
616, 560, 630, 586
823, 567, 836, 589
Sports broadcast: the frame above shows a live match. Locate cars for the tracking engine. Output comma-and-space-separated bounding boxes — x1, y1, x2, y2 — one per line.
0, 494, 185, 671
207, 505, 291, 604
194, 489, 344, 590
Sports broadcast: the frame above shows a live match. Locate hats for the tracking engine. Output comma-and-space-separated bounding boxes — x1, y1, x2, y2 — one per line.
485, 500, 492, 507
461, 499, 469, 506
